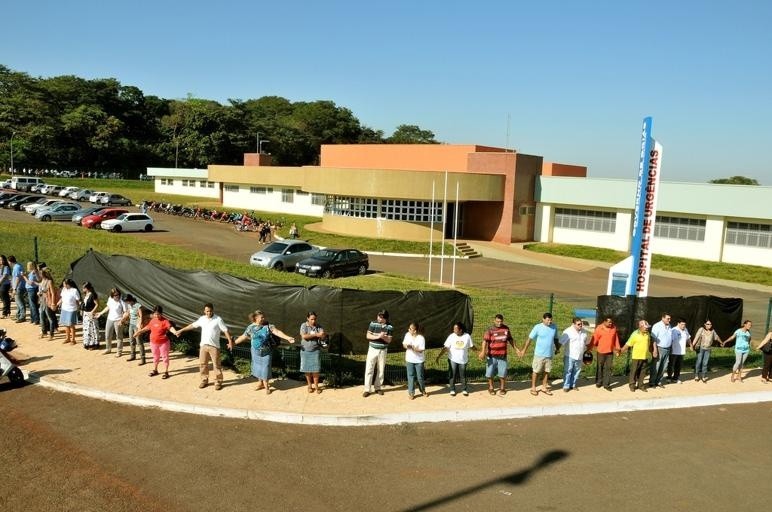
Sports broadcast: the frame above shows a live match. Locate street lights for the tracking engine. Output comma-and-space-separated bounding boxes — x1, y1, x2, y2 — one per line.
10, 131, 17, 175
260, 140, 270, 153
257, 131, 264, 153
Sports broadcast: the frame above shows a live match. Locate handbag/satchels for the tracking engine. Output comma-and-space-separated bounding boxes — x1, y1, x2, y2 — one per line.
694, 345, 700, 352
760, 339, 772, 355
316, 327, 330, 349
258, 338, 274, 357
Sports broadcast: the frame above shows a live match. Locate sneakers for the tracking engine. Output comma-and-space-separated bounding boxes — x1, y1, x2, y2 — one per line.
694, 376, 707, 383
628, 378, 683, 393
561, 387, 579, 393
0, 312, 100, 352
375, 389, 384, 396
363, 391, 369, 397
449, 390, 469, 397
215, 383, 221, 390
199, 382, 208, 387
102, 351, 146, 367
487, 388, 507, 395
407, 390, 429, 400
255, 385, 271, 395
730, 375, 742, 383
595, 383, 612, 392
760, 376, 770, 384
307, 387, 322, 394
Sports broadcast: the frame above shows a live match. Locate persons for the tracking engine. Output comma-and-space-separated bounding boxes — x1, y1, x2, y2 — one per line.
436, 322, 482, 396
226, 310, 296, 395
141, 200, 272, 245
618, 311, 694, 393
721, 320, 757, 383
133, 304, 181, 380
480, 314, 521, 395
362, 309, 394, 397
402, 321, 428, 400
554, 317, 592, 392
691, 319, 723, 382
0, 254, 147, 367
755, 331, 772, 383
176, 303, 233, 391
289, 222, 298, 240
588, 317, 621, 389
300, 311, 326, 394
520, 313, 560, 395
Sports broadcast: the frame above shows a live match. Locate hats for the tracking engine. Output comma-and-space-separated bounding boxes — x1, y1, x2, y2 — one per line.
42, 267, 53, 275
638, 320, 652, 330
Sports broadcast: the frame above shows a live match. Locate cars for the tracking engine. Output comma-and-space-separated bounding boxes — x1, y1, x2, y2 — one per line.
249, 239, 322, 273
1, 175, 154, 234
295, 246, 369, 279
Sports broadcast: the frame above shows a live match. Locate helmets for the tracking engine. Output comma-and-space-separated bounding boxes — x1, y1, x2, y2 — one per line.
582, 350, 595, 366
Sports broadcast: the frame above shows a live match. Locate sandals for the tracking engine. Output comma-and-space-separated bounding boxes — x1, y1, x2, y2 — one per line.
148, 370, 170, 379
529, 388, 552, 397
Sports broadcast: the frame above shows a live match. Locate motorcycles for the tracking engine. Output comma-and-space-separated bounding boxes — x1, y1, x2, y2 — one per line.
145, 199, 258, 232
0, 329, 23, 385
